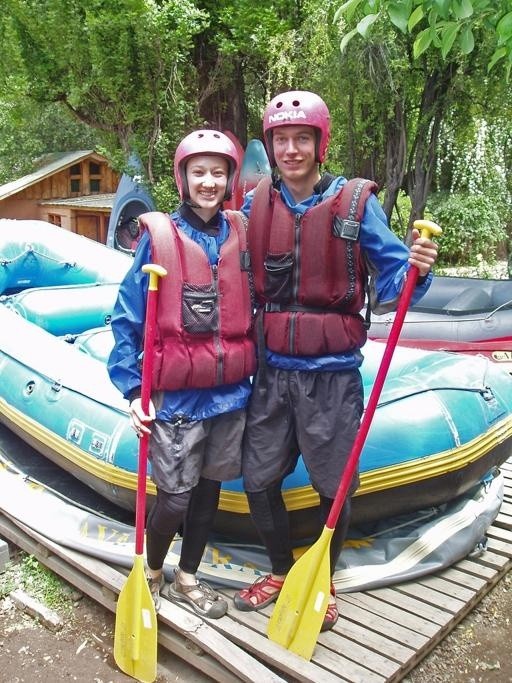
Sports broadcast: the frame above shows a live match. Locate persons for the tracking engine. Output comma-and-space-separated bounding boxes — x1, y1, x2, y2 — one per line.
234, 90, 439, 631
106, 128, 252, 618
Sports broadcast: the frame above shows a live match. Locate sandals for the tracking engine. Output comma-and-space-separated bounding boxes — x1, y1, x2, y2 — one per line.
321, 583, 338, 632
234, 574, 284, 611
168, 568, 227, 618
147, 571, 165, 610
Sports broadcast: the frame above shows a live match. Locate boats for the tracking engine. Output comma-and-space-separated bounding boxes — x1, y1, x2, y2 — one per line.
360, 263, 511, 353
223, 139, 274, 212
107, 149, 162, 256
0, 218, 511, 542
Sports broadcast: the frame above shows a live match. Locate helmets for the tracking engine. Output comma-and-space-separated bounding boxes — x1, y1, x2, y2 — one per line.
263, 91, 330, 168
174, 130, 240, 202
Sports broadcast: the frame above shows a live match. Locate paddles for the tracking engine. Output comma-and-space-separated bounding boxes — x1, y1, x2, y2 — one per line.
269, 217, 445, 661
113, 265, 171, 681
